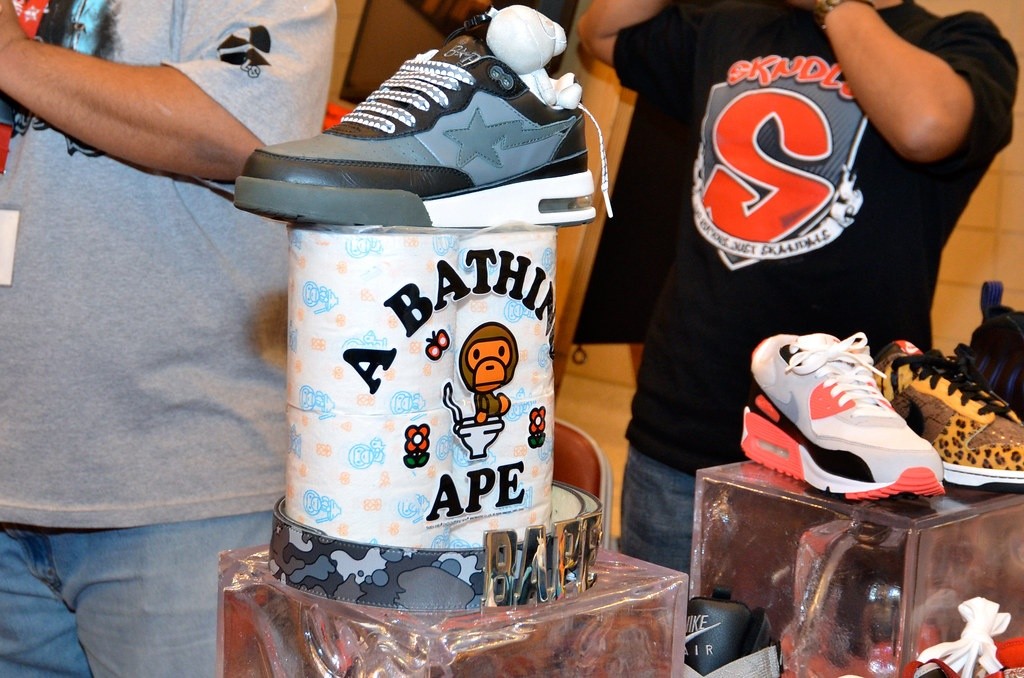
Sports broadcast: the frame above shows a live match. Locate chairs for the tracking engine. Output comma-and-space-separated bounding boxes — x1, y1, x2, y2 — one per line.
552, 419, 611, 550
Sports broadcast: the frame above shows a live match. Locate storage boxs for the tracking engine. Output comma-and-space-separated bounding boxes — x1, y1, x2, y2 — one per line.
688, 458, 1024, 677
214, 543, 689, 677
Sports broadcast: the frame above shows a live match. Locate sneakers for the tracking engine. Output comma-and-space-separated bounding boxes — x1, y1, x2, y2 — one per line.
903, 596, 1023, 677
234, 8, 595, 230
741, 281, 1024, 503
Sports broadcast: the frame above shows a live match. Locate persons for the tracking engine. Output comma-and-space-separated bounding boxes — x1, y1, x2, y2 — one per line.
577, 0, 1020, 572
0, 0, 337, 678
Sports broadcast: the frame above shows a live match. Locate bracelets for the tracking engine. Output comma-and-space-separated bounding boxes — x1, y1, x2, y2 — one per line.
813, 0, 875, 29
